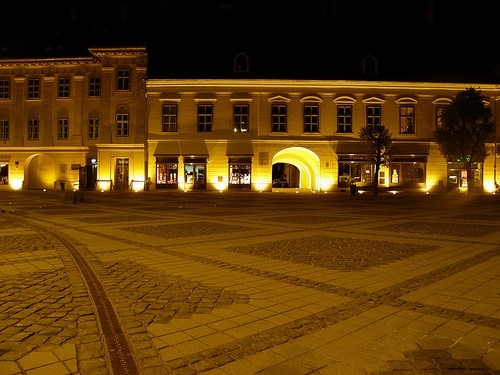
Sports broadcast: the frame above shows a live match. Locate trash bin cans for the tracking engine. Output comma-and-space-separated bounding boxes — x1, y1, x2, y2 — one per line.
53, 179, 65, 191
350, 181, 357, 194
